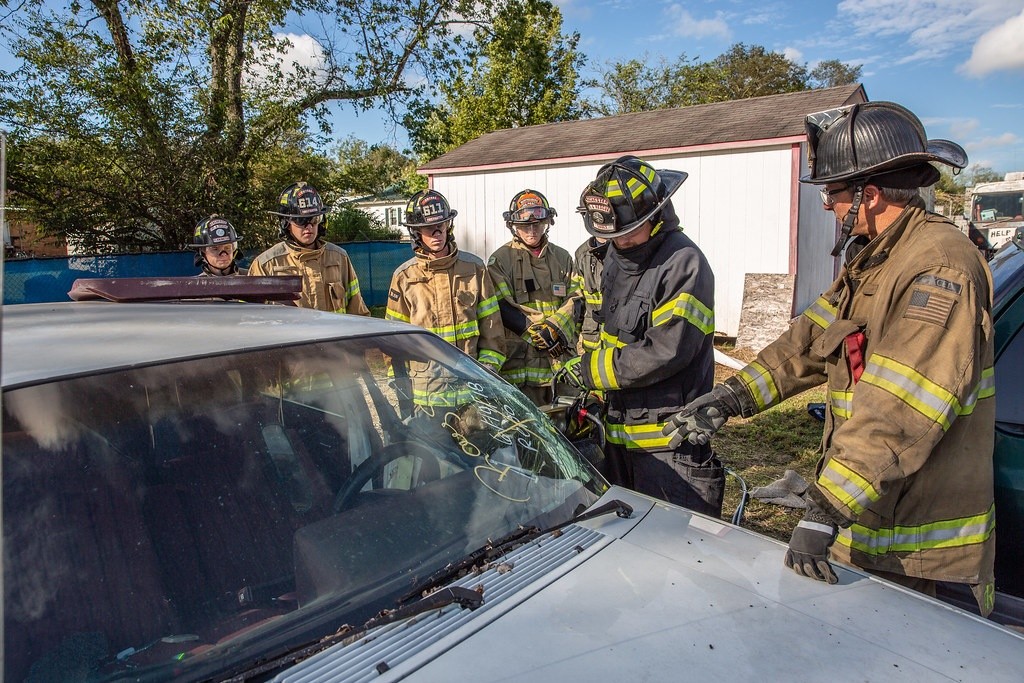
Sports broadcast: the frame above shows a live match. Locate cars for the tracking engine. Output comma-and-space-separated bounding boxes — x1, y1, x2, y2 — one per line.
937, 225, 1024, 631
0, 275, 1024, 683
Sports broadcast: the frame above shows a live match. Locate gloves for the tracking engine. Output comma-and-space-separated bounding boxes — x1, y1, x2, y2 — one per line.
784, 497, 839, 584
662, 382, 741, 450
529, 322, 560, 350
554, 356, 590, 391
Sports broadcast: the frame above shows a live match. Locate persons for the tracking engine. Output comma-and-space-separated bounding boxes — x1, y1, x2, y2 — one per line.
381, 189, 506, 433
555, 153, 725, 520
190, 215, 249, 277
246, 182, 372, 402
486, 188, 586, 470
573, 181, 612, 352
663, 102, 996, 621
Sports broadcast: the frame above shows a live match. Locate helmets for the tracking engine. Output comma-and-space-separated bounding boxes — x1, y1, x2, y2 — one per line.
798, 101, 968, 185
400, 189, 457, 226
576, 181, 594, 212
186, 214, 244, 247
267, 180, 331, 218
503, 190, 554, 226
583, 155, 689, 239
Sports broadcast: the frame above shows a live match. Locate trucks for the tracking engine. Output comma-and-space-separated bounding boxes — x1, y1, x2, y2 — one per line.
965, 170, 1024, 261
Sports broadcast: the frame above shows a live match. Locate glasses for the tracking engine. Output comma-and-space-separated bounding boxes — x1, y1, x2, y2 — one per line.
197, 242, 238, 258
285, 214, 323, 226
511, 206, 549, 221
412, 220, 450, 238
819, 180, 883, 205
